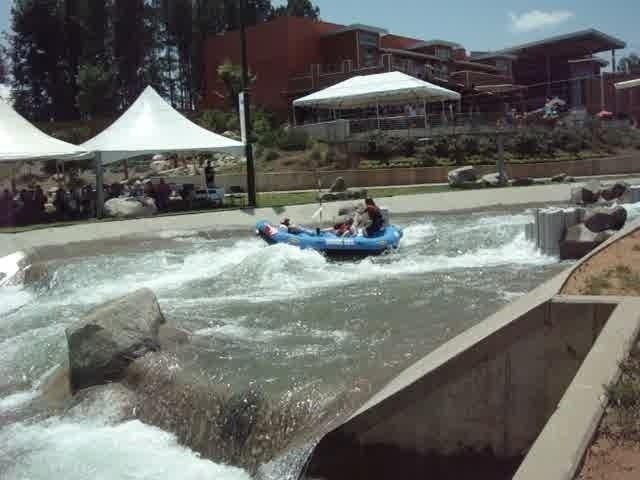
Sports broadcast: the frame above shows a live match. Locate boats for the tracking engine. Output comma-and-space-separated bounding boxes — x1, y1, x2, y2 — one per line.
257, 216, 406, 257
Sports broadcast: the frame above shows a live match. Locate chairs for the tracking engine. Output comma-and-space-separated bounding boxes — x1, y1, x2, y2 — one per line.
230, 185, 245, 204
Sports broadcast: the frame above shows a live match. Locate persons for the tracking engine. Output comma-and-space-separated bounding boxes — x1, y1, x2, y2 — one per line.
156, 177, 173, 214
281, 218, 314, 234
204, 161, 218, 189
333, 196, 384, 238
